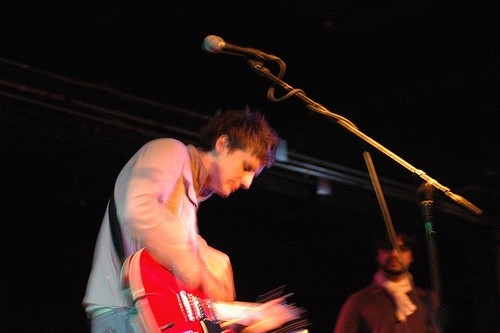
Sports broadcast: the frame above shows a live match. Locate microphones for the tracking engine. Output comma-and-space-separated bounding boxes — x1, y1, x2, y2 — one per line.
418, 182, 437, 251
203, 34, 267, 61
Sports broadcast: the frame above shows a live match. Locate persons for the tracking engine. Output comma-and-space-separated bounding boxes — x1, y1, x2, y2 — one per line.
332, 231, 442, 333
82, 110, 297, 333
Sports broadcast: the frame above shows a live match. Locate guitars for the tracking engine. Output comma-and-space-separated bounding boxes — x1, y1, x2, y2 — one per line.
120, 248, 259, 333
